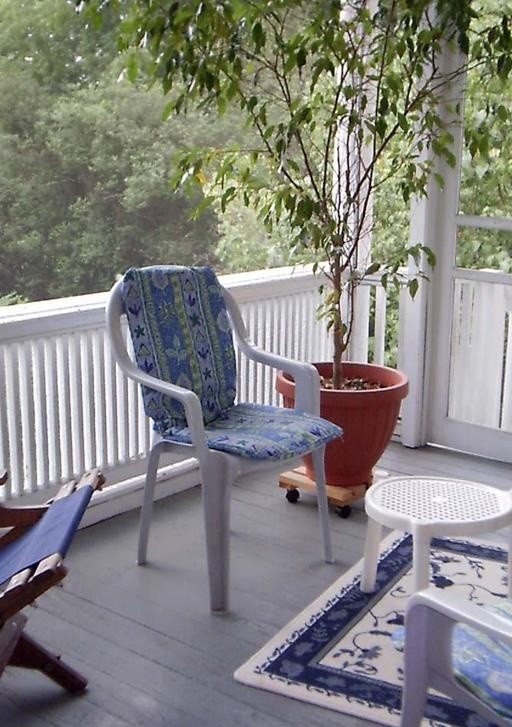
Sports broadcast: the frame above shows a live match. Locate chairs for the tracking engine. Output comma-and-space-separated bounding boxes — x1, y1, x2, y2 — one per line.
104, 266, 344, 612
0, 468, 107, 696
398, 586, 512, 727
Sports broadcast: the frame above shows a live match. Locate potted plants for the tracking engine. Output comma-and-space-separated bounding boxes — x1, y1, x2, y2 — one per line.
71, 0, 512, 487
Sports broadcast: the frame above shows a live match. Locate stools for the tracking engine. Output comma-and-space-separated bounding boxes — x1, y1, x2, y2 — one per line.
359, 475, 511, 602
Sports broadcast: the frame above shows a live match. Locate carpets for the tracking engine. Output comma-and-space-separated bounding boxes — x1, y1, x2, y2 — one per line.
233, 529, 512, 726
